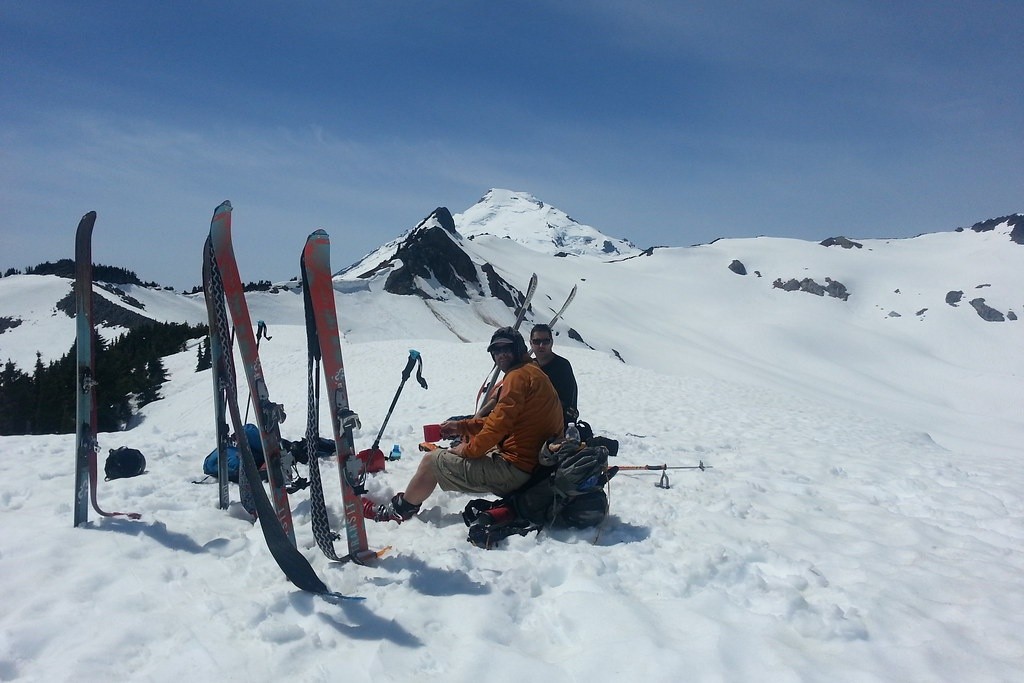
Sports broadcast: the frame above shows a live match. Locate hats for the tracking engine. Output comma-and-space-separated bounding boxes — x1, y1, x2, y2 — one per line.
487, 337, 515, 352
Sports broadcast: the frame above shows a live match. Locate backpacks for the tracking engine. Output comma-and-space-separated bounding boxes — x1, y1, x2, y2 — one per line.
515, 422, 624, 530
204, 423, 266, 484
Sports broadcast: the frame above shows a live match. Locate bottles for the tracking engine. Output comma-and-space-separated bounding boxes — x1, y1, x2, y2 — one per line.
389, 445, 401, 461
566, 422, 581, 443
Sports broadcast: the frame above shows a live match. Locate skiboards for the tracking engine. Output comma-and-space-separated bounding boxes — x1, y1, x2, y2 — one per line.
210, 199, 369, 582
480, 273, 577, 408
74, 211, 230, 527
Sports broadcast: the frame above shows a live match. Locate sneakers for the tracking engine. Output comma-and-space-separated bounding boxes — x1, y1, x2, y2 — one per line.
364, 492, 420, 526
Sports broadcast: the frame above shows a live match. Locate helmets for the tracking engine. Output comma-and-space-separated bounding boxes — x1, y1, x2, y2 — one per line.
104, 446, 146, 481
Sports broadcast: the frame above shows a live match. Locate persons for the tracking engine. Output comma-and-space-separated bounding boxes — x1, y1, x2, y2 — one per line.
390, 327, 563, 523
529, 324, 579, 427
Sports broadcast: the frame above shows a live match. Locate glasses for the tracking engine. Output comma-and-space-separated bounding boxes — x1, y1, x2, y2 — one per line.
531, 338, 553, 345
490, 345, 514, 354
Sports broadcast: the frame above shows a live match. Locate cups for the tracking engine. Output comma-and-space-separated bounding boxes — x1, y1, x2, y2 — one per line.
424, 424, 441, 442
